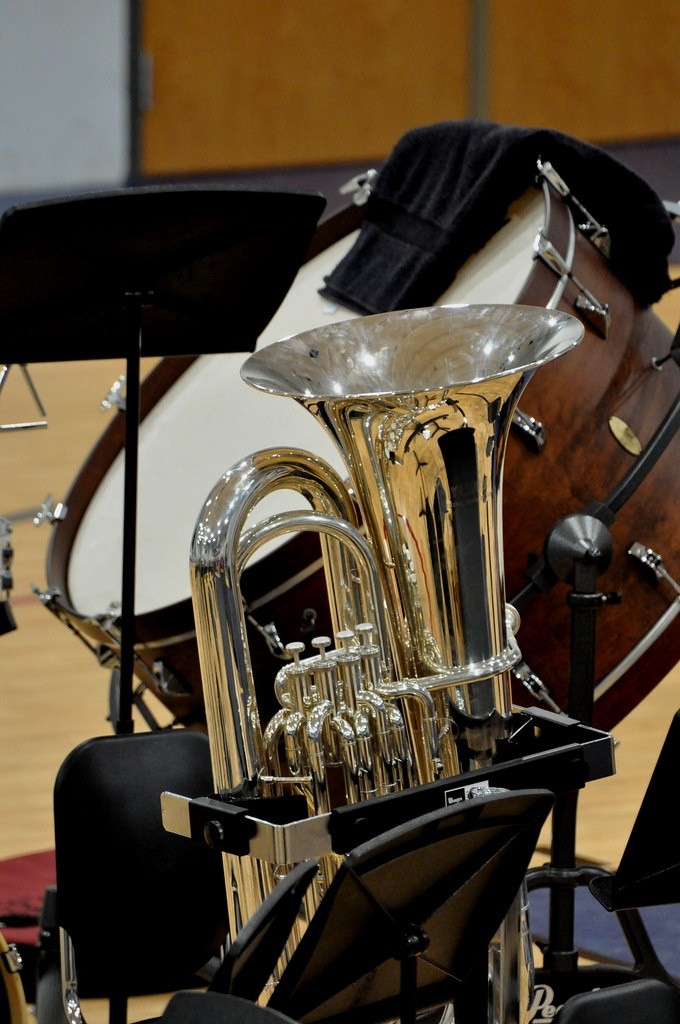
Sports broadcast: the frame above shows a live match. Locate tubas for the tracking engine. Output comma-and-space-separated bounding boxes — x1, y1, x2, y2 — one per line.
188, 303, 587, 1024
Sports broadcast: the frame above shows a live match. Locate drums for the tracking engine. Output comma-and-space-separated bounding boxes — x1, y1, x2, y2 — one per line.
29, 152, 680, 732
